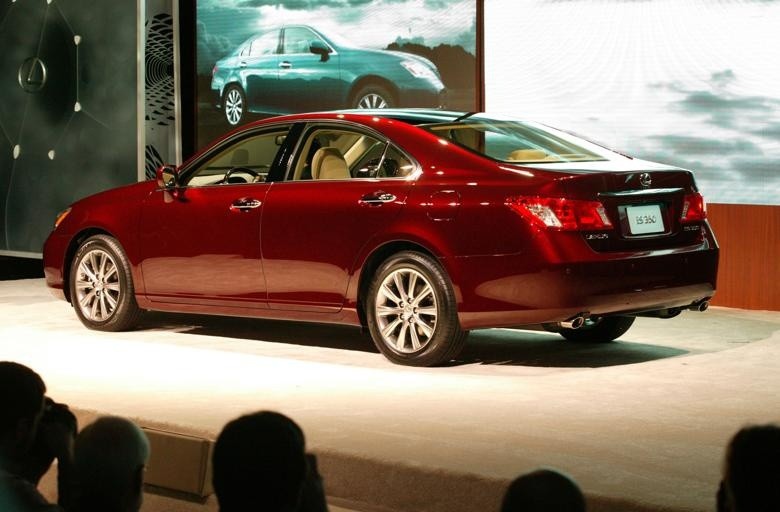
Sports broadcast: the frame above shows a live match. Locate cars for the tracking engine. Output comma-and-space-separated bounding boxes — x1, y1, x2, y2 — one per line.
208, 21, 448, 128
41, 105, 720, 365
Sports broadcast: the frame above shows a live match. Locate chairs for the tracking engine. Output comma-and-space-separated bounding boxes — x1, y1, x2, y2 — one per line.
310, 145, 353, 180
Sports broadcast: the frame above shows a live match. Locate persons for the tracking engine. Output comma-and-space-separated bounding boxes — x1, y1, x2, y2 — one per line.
499, 466, 590, 512
0, 361, 78, 511
211, 411, 332, 512
71, 416, 152, 512
715, 422, 780, 512
